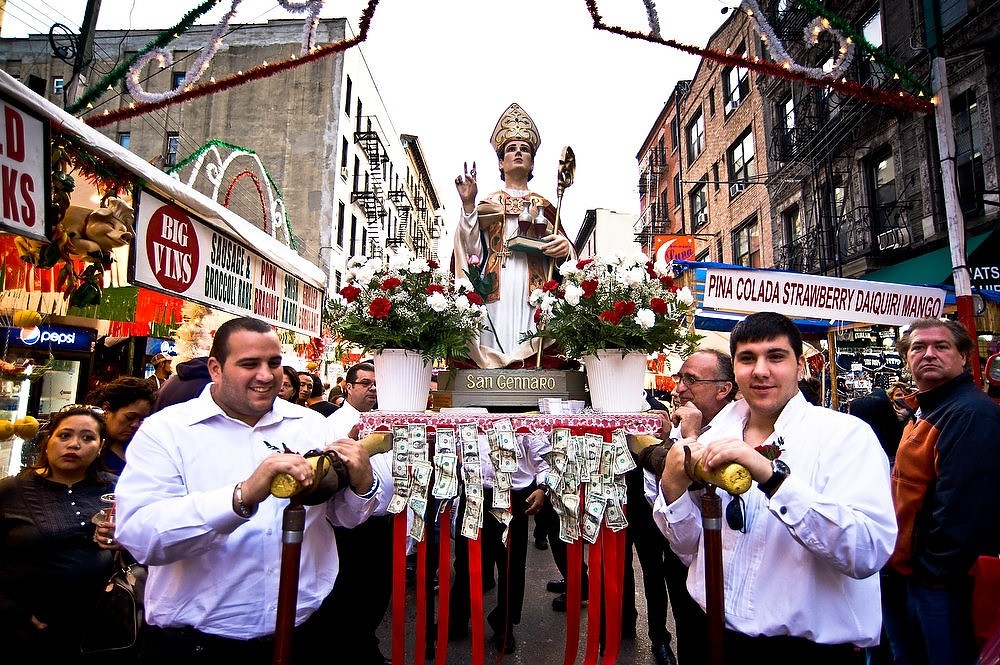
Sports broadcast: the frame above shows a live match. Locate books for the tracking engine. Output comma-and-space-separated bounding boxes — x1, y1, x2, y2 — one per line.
507, 235, 552, 258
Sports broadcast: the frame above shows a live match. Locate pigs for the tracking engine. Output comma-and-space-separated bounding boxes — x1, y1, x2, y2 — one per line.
14, 196, 141, 270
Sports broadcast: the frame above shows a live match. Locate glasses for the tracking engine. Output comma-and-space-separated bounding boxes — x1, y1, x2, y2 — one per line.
670, 373, 729, 389
351, 380, 376, 388
57, 402, 104, 420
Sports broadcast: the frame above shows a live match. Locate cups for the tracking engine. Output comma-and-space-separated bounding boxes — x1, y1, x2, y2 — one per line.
100, 493, 116, 523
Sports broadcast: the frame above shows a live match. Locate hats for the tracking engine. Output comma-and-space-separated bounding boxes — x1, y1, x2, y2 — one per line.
150, 353, 173, 366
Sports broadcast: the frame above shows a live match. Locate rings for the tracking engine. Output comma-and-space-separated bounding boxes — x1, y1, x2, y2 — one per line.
106, 538, 112, 544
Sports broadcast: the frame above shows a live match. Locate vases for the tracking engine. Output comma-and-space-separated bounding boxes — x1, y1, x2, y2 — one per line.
581, 345, 650, 414
515, 201, 549, 239
370, 349, 433, 416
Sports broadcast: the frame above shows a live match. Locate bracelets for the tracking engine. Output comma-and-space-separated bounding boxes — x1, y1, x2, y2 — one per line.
238, 482, 253, 518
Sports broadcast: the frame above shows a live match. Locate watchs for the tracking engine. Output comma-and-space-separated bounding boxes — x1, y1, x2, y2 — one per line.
758, 459, 790, 496
357, 471, 379, 498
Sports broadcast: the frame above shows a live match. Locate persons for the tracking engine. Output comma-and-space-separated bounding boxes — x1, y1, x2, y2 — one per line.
453, 135, 575, 368
402, 376, 678, 665
670, 345, 740, 439
890, 318, 999, 664
0, 407, 122, 663
798, 376, 822, 406
87, 352, 378, 495
114, 318, 381, 664
653, 311, 902, 665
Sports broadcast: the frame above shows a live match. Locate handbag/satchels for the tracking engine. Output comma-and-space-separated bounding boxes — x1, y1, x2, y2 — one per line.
73, 544, 149, 659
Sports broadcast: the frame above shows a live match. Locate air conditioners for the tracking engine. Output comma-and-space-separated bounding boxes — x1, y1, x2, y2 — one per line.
730, 182, 745, 197
341, 166, 349, 179
698, 213, 708, 226
724, 100, 738, 116
373, 247, 383, 257
877, 229, 903, 250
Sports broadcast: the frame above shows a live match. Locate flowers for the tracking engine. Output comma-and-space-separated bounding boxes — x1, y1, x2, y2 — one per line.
461, 253, 507, 356
754, 435, 788, 461
516, 252, 706, 363
320, 244, 493, 369
263, 439, 301, 455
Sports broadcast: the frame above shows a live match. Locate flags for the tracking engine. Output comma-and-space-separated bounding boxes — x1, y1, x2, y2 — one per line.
655, 234, 696, 278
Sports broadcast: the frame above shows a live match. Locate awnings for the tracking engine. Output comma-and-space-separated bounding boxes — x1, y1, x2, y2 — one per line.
669, 260, 999, 331
859, 232, 993, 285
